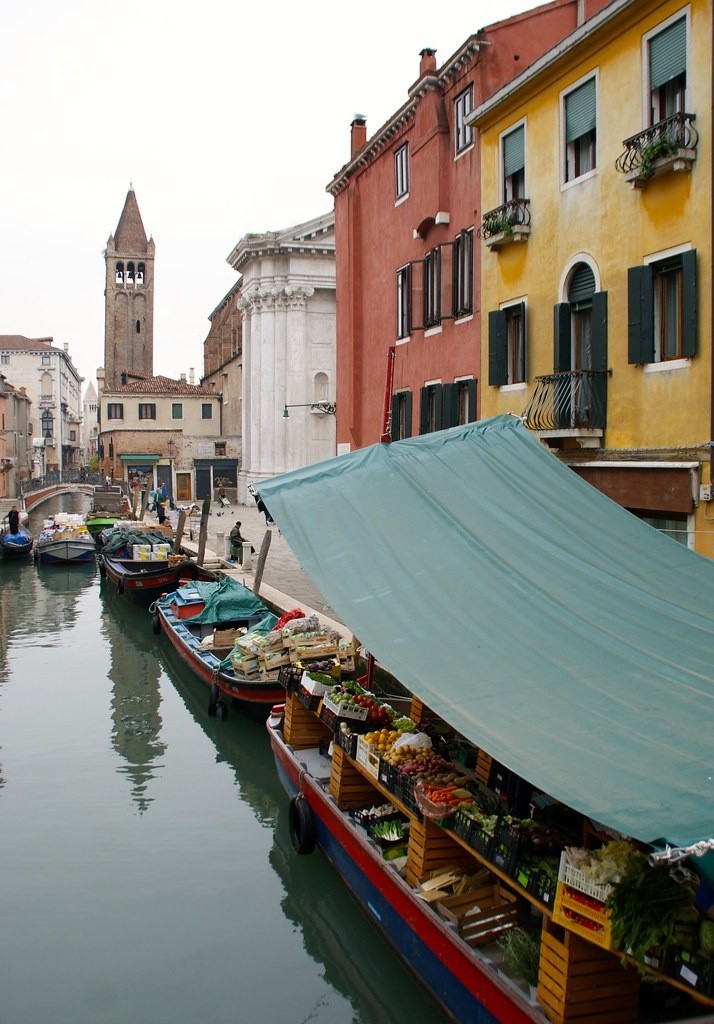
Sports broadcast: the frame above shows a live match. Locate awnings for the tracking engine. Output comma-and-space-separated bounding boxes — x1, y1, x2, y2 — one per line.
120, 455, 159, 462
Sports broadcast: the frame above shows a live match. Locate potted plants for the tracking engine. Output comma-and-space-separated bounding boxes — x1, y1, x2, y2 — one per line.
483, 211, 522, 238
640, 132, 679, 180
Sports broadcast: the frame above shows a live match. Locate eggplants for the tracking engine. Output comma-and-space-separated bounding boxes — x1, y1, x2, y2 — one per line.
508, 816, 567, 849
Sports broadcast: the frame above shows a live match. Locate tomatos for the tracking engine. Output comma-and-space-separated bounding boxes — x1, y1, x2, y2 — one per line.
328, 688, 392, 728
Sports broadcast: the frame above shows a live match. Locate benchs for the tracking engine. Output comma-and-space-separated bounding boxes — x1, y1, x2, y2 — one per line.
229, 546, 243, 565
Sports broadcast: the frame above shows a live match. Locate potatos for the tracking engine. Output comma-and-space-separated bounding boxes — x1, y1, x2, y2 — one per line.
382, 744, 471, 788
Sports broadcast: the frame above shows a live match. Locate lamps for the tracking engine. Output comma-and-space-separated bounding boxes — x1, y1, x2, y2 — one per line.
282, 402, 336, 420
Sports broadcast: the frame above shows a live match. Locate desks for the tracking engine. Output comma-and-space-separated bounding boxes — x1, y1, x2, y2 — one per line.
284, 689, 387, 811
406, 816, 645, 1024
327, 743, 475, 888
536, 912, 714, 1024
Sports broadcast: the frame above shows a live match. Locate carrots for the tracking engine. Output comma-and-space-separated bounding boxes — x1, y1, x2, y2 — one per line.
426, 786, 474, 806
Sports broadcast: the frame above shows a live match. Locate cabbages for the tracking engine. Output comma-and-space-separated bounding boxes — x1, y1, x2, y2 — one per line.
679, 919, 714, 989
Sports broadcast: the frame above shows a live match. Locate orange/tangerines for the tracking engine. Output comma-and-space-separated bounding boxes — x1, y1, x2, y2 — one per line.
364, 728, 403, 750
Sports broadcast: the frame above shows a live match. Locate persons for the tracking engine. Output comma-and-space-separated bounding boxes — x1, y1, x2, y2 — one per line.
129, 479, 138, 506
3, 506, 21, 536
153, 483, 174, 523
218, 481, 226, 508
230, 521, 248, 564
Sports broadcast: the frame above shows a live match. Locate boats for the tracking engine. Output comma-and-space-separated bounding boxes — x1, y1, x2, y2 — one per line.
264, 695, 714, 1024
33, 512, 97, 561
1, 523, 33, 554
152, 582, 345, 704
98, 521, 221, 597
84, 477, 133, 540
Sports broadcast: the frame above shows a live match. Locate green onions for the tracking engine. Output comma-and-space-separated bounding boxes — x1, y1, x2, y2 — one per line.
370, 820, 407, 841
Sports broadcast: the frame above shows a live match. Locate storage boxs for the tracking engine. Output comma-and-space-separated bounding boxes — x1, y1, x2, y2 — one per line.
40, 513, 714, 998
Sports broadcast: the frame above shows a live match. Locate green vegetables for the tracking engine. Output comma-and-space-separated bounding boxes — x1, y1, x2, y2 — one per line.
594, 853, 702, 973
455, 810, 543, 861
521, 855, 560, 894
341, 681, 417, 732
306, 671, 337, 686
